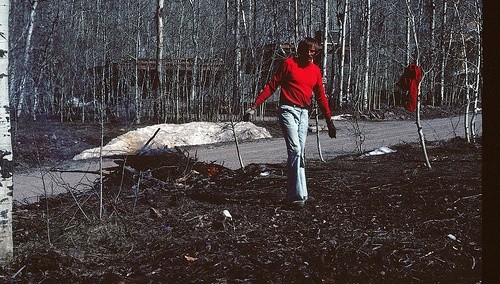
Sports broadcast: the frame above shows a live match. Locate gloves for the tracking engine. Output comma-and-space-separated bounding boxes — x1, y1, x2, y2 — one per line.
325, 119, 336, 139
243, 104, 257, 122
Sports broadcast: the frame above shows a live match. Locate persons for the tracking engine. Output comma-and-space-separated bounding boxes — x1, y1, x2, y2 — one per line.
243, 37, 336, 209
